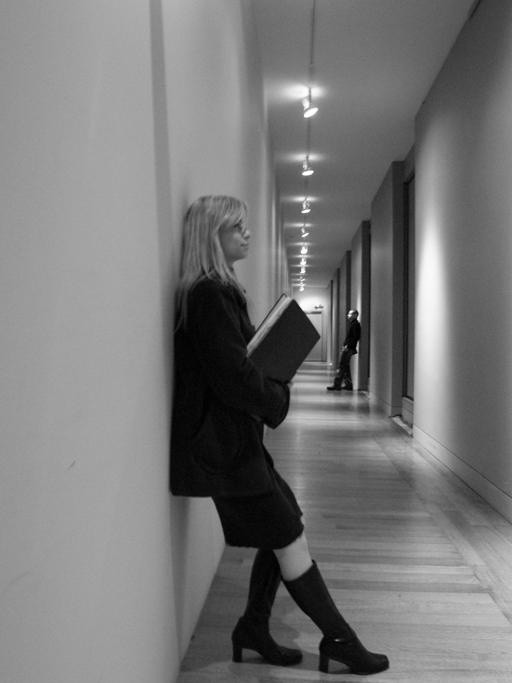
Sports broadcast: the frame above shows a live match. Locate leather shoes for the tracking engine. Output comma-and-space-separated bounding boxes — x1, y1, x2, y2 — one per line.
326, 384, 354, 390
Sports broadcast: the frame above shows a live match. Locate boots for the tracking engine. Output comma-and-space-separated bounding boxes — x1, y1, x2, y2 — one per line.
229, 546, 304, 667
284, 558, 390, 674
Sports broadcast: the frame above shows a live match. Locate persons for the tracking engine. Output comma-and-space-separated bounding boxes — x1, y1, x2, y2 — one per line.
170, 193, 388, 675
325, 308, 361, 392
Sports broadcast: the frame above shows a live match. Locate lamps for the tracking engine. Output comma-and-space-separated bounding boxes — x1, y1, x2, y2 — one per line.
298, 96, 318, 292
